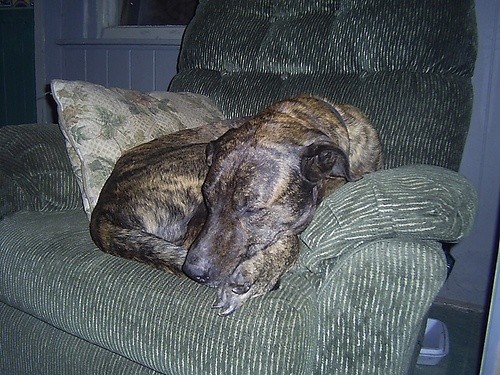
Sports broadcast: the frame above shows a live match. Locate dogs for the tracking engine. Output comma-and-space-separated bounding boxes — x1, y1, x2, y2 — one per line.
90, 95, 386, 316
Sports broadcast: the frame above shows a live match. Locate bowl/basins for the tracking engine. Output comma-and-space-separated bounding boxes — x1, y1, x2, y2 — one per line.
416, 318, 450, 366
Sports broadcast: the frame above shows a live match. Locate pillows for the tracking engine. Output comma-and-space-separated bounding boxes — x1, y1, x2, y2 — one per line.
48, 78, 226, 226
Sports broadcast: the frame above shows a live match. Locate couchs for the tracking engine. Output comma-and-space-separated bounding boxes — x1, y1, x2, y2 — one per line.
0, 0, 481, 373
